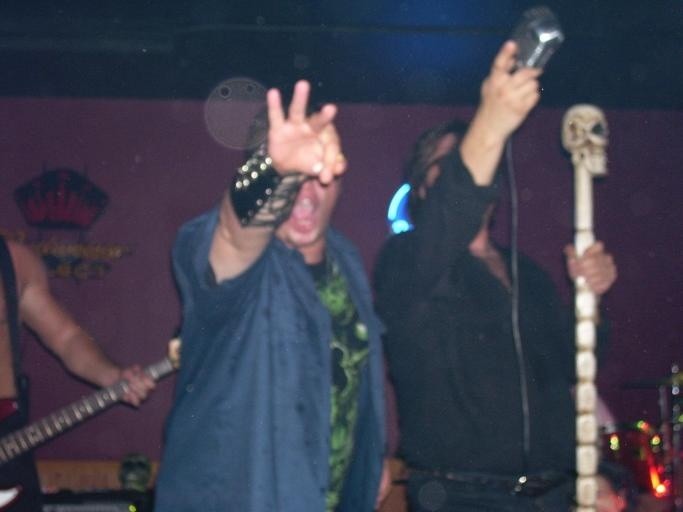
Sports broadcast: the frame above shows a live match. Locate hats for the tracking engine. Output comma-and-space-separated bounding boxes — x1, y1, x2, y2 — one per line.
412, 118, 469, 172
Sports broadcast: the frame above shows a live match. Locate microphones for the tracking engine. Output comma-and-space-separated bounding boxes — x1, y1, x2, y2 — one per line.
507, 7, 564, 77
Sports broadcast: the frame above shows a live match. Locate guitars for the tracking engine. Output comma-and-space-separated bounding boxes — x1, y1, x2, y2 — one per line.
0, 335, 183, 511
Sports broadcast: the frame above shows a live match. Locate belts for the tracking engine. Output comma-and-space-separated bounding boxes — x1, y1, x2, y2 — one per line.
409, 469, 566, 500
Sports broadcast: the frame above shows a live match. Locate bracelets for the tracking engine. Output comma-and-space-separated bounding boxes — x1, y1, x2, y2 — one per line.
229, 145, 307, 229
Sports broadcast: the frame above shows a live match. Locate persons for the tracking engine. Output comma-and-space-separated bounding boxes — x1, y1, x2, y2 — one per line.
0, 234, 155, 511
372, 35, 618, 511
152, 80, 392, 512
593, 462, 638, 512
